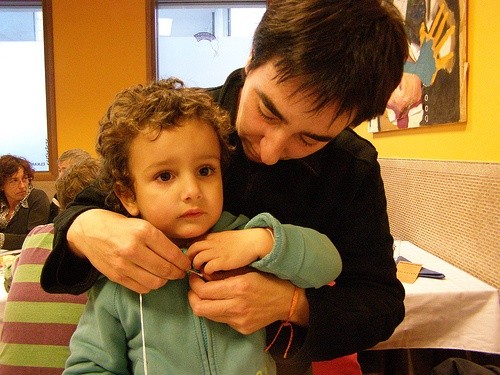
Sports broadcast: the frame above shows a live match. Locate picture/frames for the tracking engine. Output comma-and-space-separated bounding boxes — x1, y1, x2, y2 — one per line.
370, 0, 470, 139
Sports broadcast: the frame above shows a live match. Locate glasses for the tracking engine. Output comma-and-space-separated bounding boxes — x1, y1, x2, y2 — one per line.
5, 176, 32, 186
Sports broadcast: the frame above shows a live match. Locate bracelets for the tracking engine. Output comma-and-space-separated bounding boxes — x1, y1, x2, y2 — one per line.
266, 227, 275, 249
263, 286, 299, 359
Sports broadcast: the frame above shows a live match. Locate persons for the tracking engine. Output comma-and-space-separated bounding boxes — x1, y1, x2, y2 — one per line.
0, 154, 51, 251
62, 77, 343, 375
40, 0, 409, 375
0, 148, 105, 375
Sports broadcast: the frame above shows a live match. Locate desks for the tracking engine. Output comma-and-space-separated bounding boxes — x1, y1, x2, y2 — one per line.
358, 240, 499, 375
